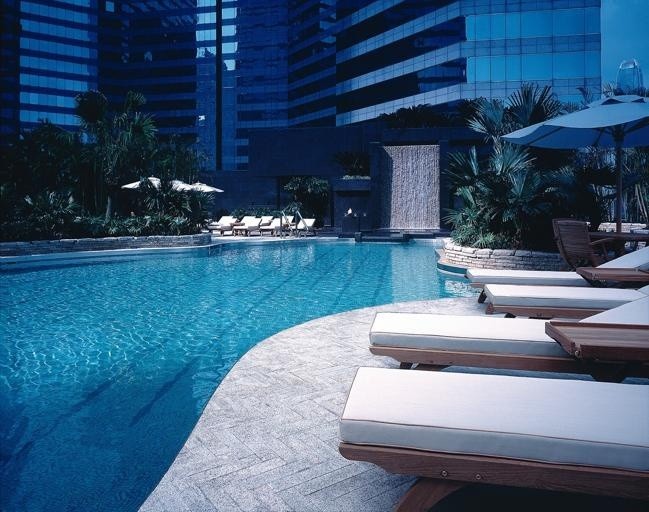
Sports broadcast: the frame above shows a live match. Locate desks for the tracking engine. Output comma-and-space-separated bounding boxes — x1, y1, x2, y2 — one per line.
545, 319, 647, 360
589, 230, 648, 258
575, 267, 648, 288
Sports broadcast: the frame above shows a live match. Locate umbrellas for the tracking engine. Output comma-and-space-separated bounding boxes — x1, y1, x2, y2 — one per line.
120, 175, 225, 220
498, 93, 649, 233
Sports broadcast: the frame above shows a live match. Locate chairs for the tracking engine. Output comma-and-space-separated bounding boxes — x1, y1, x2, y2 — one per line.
467, 246, 648, 305
339, 364, 647, 512
552, 218, 622, 270
207, 215, 317, 238
366, 296, 649, 384
483, 284, 648, 319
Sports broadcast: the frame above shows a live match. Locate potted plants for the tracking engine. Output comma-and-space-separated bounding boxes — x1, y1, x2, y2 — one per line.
332, 151, 370, 176
